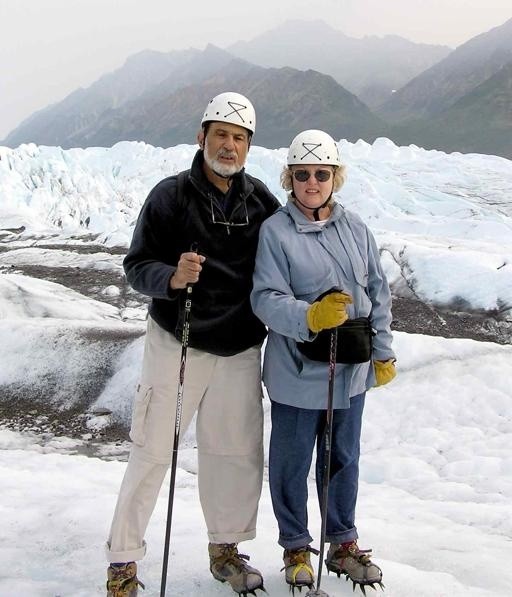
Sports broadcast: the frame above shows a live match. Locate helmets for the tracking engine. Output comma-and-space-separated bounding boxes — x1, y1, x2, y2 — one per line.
200, 91, 256, 137
287, 129, 341, 168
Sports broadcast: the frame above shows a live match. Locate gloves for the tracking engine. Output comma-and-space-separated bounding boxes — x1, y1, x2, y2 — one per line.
373, 358, 398, 388
305, 289, 353, 334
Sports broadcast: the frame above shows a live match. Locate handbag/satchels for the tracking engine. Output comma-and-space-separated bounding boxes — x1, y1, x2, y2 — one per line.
295, 285, 374, 365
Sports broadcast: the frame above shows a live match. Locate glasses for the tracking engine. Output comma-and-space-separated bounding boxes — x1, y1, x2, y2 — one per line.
293, 169, 332, 183
209, 195, 250, 232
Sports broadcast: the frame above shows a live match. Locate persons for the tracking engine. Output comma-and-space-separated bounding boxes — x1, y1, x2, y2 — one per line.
105, 91, 282, 596
252, 126, 396, 586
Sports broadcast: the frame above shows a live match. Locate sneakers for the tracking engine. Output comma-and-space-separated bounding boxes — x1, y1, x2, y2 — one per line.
208, 541, 263, 593
282, 544, 316, 585
105, 560, 139, 597
324, 538, 382, 585
